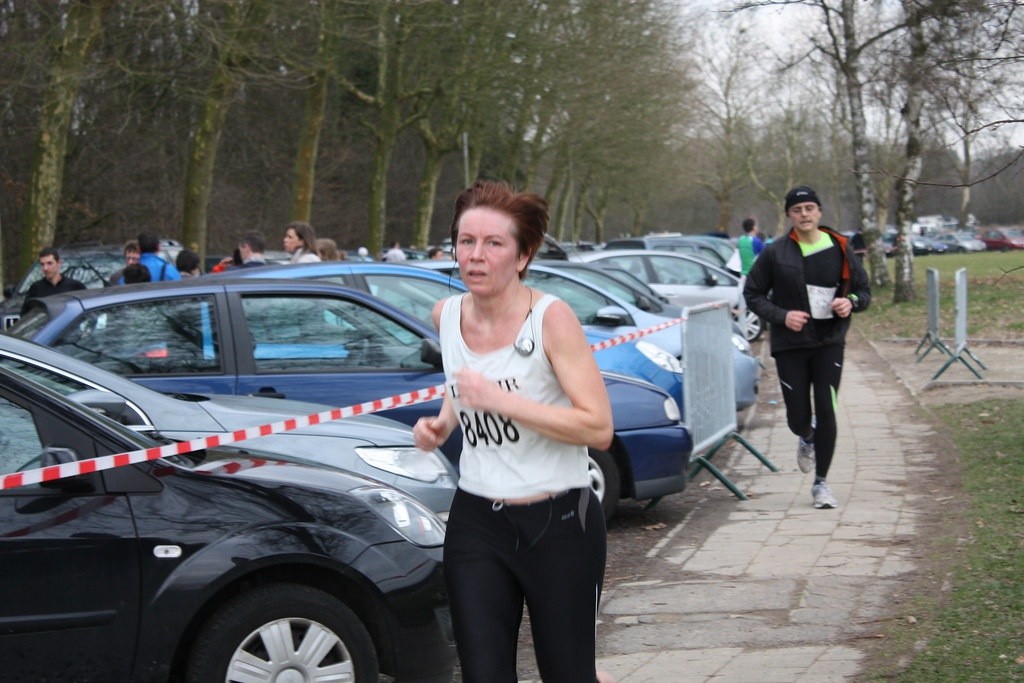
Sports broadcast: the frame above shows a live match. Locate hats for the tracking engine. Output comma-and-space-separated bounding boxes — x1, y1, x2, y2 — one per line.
784, 186, 822, 211
176, 250, 201, 271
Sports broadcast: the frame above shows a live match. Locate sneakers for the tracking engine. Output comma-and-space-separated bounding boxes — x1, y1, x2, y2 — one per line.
796, 415, 816, 473
811, 481, 838, 509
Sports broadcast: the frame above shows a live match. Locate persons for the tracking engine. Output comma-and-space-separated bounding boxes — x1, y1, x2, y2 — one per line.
412, 181, 614, 683
381, 241, 444, 264
737, 217, 775, 277
105, 222, 339, 330
849, 227, 866, 261
23, 246, 97, 346
743, 186, 873, 509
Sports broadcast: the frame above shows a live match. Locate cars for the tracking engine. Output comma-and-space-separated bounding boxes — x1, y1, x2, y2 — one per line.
0, 212, 1024, 533
1, 327, 463, 683
